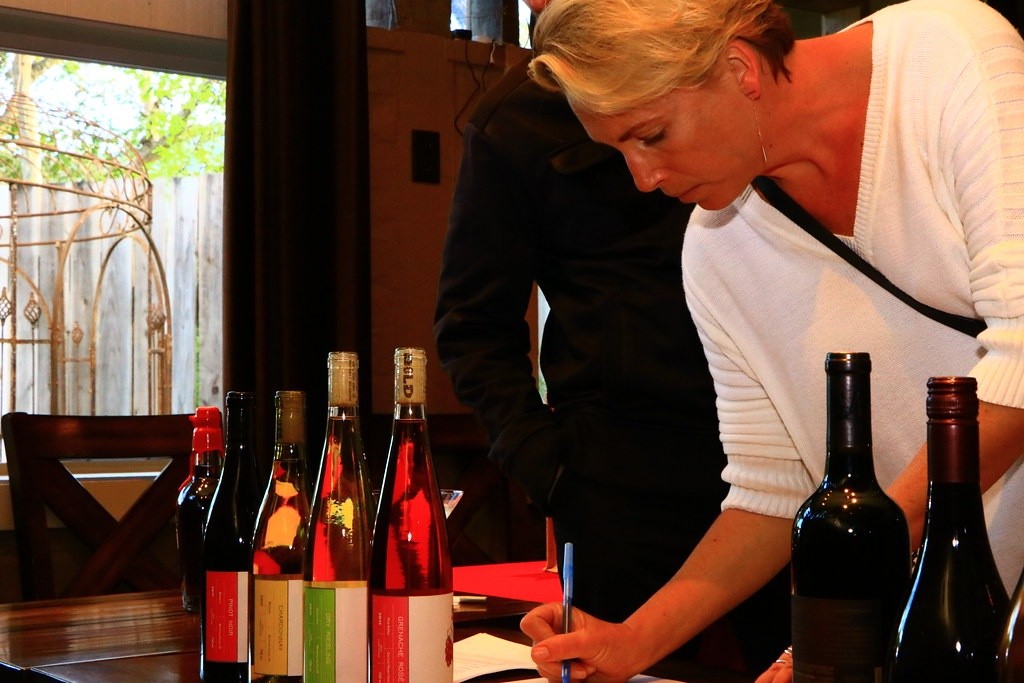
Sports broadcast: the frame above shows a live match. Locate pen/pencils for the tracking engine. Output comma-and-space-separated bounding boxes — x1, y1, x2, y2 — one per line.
561, 541, 575, 682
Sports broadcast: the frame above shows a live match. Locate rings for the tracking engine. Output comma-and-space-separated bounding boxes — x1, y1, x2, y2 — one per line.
784, 647, 792, 655
776, 659, 787, 663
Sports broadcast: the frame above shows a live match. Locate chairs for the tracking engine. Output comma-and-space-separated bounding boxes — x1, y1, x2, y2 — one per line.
354, 410, 548, 566
1, 412, 227, 602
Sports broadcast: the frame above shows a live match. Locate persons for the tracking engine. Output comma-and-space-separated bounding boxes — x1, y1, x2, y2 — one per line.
432, 0, 792, 683
519, 0, 1024, 683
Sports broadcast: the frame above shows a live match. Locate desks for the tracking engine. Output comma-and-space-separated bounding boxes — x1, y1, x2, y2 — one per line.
0, 585, 732, 683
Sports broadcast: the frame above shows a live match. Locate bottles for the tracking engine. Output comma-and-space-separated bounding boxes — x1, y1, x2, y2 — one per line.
789, 353, 908, 683
893, 376, 1009, 683
997, 566, 1023, 682
302, 351, 377, 683
366, 347, 454, 683
246, 391, 309, 683
200, 390, 259, 683
176, 408, 224, 612
